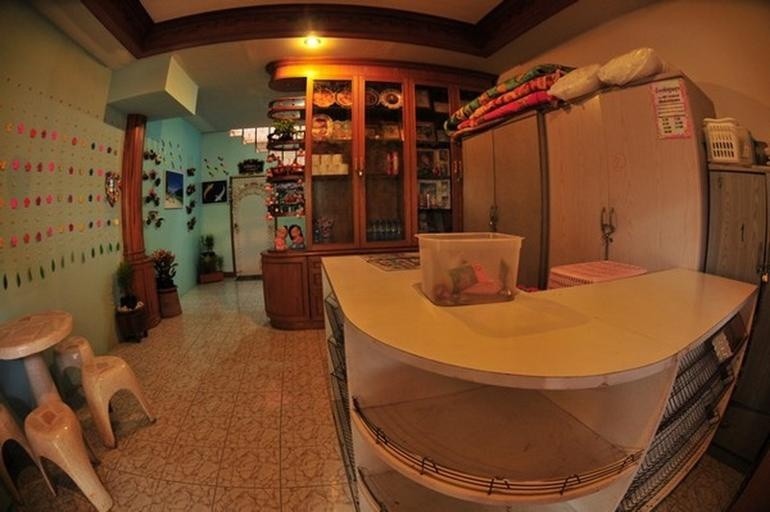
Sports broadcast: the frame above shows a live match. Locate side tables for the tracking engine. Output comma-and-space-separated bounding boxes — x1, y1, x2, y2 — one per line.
0, 310, 73, 408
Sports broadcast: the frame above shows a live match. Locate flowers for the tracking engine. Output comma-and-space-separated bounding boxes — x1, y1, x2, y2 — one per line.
282, 191, 303, 205
262, 184, 280, 221
288, 149, 305, 168
266, 153, 285, 178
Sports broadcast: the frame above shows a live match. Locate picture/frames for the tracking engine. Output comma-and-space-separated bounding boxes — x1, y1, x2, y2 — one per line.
164, 170, 184, 209
201, 180, 227, 203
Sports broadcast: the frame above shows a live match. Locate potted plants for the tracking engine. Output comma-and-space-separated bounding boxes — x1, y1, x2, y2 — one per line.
199, 234, 218, 272
151, 248, 183, 318
115, 261, 149, 344
271, 118, 297, 141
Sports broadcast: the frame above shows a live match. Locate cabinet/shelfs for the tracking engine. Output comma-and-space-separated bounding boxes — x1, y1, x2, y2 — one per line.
542, 69, 717, 272
458, 112, 547, 289
705, 160, 770, 477
260, 55, 496, 330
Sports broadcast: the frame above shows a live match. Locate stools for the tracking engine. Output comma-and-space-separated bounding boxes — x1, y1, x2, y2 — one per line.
53, 336, 113, 413
1, 403, 36, 505
81, 356, 157, 449
24, 402, 115, 512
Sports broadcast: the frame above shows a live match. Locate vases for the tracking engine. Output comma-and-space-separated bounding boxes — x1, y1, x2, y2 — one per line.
290, 168, 305, 175
284, 203, 302, 214
268, 204, 281, 216
272, 168, 287, 176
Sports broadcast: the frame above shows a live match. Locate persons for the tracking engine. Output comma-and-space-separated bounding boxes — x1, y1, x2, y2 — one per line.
289, 224, 304, 249
272, 224, 290, 252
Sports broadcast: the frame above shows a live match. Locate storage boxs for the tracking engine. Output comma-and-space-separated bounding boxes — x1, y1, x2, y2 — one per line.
415, 233, 526, 306
549, 261, 647, 289
707, 117, 757, 166
